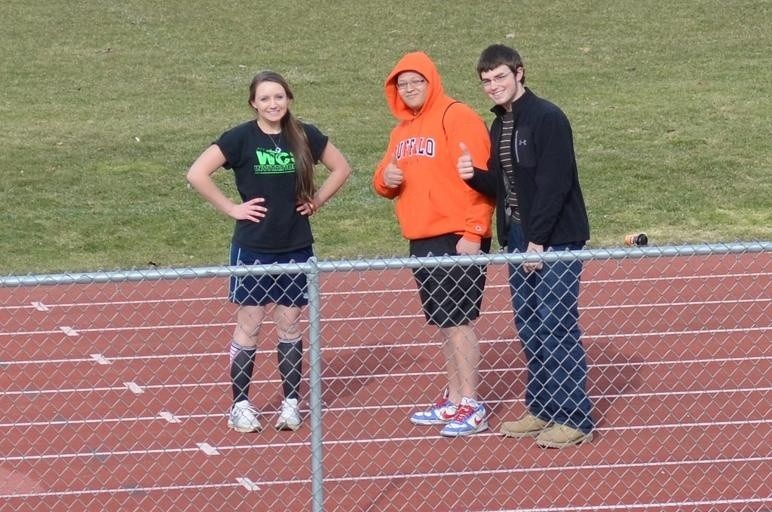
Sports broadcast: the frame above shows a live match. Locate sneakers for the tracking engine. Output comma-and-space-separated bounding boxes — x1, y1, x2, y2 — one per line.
440, 397, 488, 436
227, 400, 263, 433
275, 398, 301, 431
410, 388, 458, 425
500, 414, 553, 437
536, 423, 593, 449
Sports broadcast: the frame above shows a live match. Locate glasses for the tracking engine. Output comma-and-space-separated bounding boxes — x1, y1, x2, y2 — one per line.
481, 71, 512, 86
395, 80, 424, 90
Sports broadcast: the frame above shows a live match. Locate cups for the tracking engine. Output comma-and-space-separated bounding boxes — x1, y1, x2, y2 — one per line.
624, 233, 649, 247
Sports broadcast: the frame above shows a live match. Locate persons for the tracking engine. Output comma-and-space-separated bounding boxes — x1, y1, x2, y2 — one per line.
455, 44, 595, 449
184, 71, 354, 434
372, 51, 497, 437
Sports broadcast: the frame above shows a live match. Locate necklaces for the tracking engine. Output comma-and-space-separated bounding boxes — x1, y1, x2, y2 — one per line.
268, 133, 283, 153
